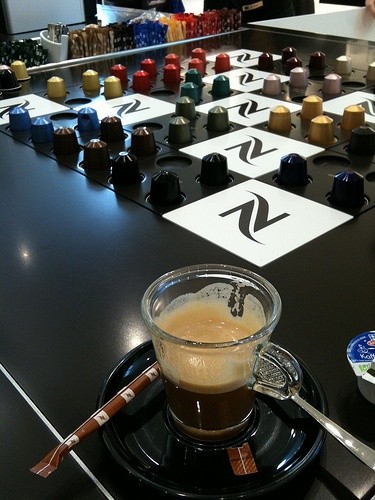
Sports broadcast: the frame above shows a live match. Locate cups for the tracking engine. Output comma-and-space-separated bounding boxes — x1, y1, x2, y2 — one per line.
139, 264, 302, 400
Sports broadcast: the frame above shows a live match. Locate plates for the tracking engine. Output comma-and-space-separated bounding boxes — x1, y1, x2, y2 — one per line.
99, 337, 327, 499
